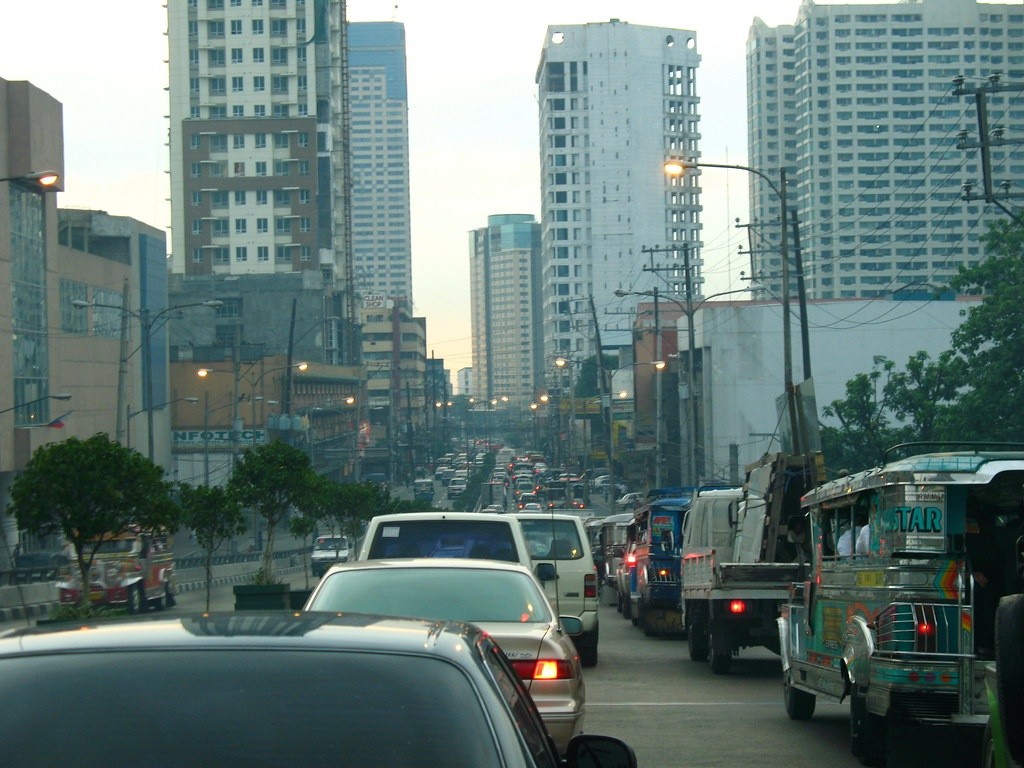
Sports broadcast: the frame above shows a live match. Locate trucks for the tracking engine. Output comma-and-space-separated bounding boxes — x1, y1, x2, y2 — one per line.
58, 525, 178, 616
660, 482, 810, 676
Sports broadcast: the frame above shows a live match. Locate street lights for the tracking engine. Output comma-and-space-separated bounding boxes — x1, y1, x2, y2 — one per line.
540, 391, 627, 501
204, 391, 264, 488
283, 315, 342, 443
664, 160, 793, 393
435, 396, 508, 481
196, 360, 308, 550
265, 395, 354, 538
555, 357, 668, 516
70, 300, 224, 466
614, 283, 766, 486
126, 396, 199, 449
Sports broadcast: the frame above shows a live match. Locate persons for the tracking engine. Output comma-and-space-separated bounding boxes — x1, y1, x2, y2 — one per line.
395, 542, 423, 557
836, 523, 862, 559
787, 520, 805, 543
856, 521, 871, 557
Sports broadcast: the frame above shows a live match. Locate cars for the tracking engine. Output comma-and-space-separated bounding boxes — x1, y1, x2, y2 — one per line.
8, 549, 65, 586
414, 439, 643, 515
0, 608, 640, 767
302, 557, 587, 754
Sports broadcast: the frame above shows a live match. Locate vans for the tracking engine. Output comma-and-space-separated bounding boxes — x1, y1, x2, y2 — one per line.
583, 485, 732, 639
773, 439, 1023, 764
311, 533, 352, 578
357, 513, 557, 591
501, 511, 605, 668
